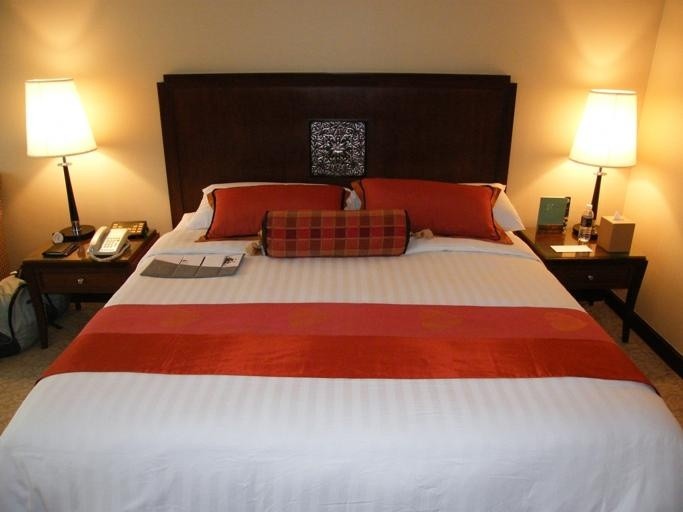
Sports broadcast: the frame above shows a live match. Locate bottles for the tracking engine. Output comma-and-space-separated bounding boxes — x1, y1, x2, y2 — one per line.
578, 203, 594, 243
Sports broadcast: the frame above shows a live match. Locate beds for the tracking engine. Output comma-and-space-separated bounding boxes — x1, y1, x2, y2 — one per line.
0, 73, 683, 512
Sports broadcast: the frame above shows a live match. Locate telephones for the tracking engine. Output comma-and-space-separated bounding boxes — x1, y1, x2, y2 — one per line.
86, 226, 128, 256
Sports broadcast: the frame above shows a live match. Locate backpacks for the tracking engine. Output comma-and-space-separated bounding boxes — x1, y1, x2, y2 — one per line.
0, 271, 60, 358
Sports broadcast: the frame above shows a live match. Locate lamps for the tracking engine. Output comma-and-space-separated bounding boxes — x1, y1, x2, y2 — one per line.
571, 88, 637, 238
25, 79, 96, 243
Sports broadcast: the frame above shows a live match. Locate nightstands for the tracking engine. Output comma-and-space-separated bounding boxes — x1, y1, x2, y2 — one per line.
517, 228, 647, 343
23, 231, 161, 349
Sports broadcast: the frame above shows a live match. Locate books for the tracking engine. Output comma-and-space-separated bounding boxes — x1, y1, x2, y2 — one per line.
41, 241, 80, 258
